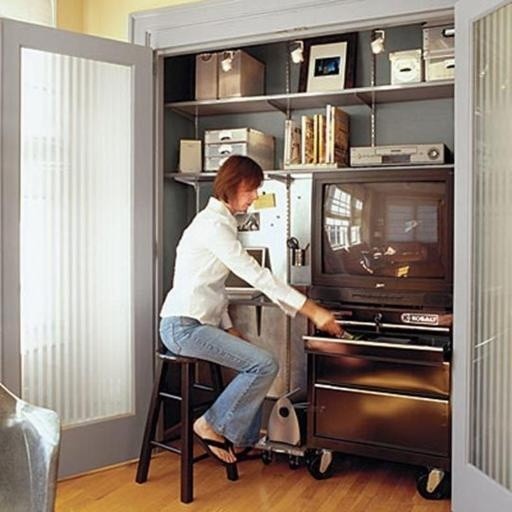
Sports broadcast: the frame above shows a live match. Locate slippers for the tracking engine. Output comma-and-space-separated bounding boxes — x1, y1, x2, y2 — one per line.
191, 430, 238, 468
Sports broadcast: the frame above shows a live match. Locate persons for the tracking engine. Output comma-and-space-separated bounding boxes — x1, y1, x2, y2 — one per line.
158, 154, 345, 464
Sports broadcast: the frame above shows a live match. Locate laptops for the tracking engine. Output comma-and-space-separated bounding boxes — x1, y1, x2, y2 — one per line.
225, 247, 266, 300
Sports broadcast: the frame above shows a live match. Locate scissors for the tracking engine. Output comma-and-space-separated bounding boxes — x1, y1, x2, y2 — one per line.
287, 238, 299, 249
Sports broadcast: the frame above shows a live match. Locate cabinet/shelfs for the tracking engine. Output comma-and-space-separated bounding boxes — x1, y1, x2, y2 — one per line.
159, 81, 450, 179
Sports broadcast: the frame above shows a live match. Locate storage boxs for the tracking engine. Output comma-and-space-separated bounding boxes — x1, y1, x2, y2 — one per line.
194, 50, 267, 100
204, 126, 276, 173
386, 21, 454, 85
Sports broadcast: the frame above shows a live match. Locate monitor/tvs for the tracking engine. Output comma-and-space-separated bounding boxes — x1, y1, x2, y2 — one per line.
311, 168, 454, 309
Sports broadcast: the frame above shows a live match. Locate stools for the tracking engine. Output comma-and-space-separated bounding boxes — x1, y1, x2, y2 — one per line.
134, 350, 242, 504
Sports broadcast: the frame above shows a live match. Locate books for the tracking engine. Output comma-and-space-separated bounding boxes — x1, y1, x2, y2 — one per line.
279, 103, 350, 171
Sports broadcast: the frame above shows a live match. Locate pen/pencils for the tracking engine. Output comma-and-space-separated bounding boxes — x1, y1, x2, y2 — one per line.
304, 244, 309, 251
